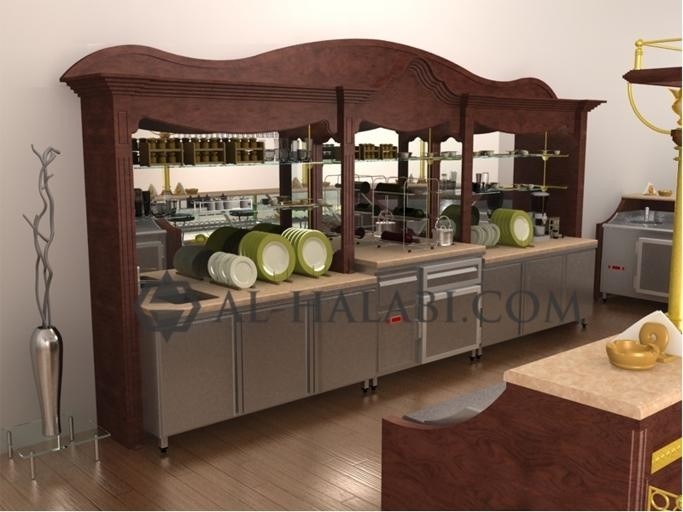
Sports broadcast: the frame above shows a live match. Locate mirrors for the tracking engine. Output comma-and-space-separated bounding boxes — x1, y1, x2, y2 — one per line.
130, 127, 516, 273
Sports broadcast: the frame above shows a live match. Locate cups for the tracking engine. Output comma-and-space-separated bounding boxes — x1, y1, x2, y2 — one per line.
527, 184, 534, 190
490, 182, 500, 189
554, 150, 561, 155
277, 196, 289, 204
480, 172, 489, 192
514, 184, 521, 189
534, 211, 547, 235
521, 150, 529, 156
398, 151, 410, 161
440, 151, 456, 158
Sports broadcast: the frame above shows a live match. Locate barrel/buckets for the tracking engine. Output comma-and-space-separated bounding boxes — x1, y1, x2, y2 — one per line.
431, 213, 453, 246
374, 208, 397, 235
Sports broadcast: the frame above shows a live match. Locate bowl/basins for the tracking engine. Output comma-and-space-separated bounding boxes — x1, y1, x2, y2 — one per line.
658, 190, 672, 198
607, 339, 660, 371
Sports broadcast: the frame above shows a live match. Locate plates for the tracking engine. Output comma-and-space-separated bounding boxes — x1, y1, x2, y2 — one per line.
169, 222, 334, 290
441, 204, 535, 249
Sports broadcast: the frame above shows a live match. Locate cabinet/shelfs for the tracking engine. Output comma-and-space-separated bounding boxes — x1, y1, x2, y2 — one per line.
152, 183, 341, 264
133, 214, 167, 271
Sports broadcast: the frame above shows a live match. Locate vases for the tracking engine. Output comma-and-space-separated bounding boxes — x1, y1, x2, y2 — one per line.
29, 326, 63, 437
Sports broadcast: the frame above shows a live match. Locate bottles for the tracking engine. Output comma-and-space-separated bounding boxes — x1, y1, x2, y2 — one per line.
440, 172, 449, 194
131, 134, 266, 164
364, 143, 397, 159
333, 181, 428, 245
134, 188, 143, 217
184, 191, 251, 212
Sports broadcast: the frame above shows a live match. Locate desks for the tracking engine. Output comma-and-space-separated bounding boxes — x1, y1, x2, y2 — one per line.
2, 410, 111, 481
